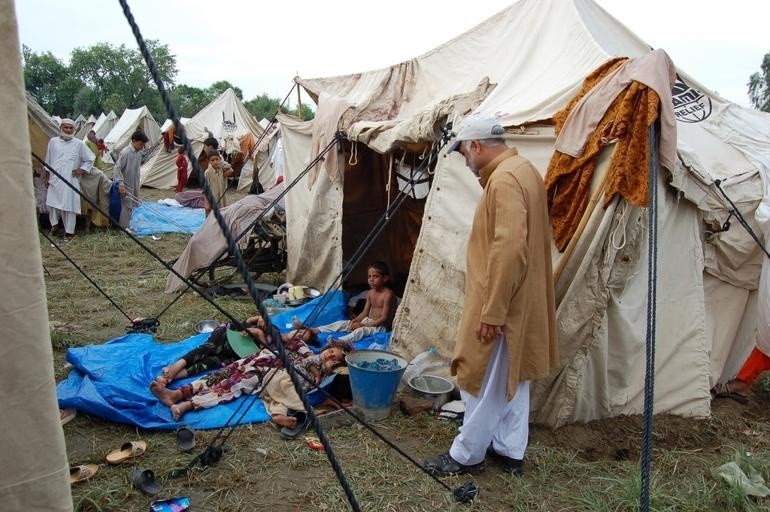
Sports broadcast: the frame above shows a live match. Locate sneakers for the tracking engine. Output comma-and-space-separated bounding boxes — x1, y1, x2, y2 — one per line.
59, 408, 76, 426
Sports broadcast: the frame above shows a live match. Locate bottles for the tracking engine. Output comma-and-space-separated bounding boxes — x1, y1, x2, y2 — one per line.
397, 347, 437, 392
414, 372, 430, 392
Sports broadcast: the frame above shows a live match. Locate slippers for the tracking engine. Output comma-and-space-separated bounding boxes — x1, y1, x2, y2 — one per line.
106, 440, 147, 465
128, 468, 164, 496
69, 464, 99, 484
315, 406, 367, 430
175, 425, 196, 452
280, 409, 310, 441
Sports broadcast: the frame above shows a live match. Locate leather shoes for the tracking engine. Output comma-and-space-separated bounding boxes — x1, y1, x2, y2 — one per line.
424, 451, 486, 477
486, 444, 524, 474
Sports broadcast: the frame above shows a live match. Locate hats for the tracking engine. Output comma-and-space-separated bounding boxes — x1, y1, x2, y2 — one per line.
61, 118, 76, 128
445, 115, 507, 155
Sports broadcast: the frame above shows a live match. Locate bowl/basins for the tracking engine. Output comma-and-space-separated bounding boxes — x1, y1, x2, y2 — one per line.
194, 319, 220, 334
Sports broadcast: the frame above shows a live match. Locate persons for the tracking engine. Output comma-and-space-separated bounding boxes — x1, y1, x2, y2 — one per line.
149, 260, 399, 428
45, 117, 149, 239
424, 114, 564, 477
173, 137, 233, 214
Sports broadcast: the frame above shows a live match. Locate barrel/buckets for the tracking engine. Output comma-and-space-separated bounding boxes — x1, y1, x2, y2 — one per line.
343, 350, 407, 423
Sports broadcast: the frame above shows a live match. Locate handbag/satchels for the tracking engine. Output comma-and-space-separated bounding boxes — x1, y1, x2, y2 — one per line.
399, 395, 438, 417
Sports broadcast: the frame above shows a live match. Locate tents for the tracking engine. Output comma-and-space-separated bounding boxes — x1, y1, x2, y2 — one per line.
274, 1, 769, 434
50, 87, 274, 191
23, 89, 117, 214
